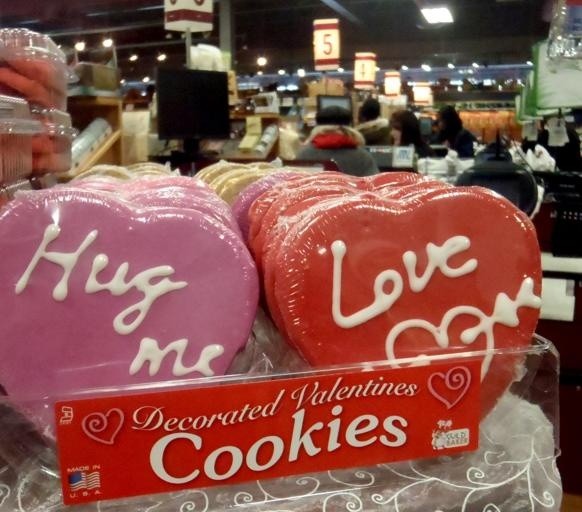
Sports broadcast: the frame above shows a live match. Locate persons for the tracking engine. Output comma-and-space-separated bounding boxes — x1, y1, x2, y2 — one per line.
427, 105, 479, 157
279, 119, 302, 161
353, 98, 394, 145
295, 105, 380, 177
388, 111, 437, 158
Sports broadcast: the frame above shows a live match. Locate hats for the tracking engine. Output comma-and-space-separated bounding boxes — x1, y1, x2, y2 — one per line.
316, 105, 352, 125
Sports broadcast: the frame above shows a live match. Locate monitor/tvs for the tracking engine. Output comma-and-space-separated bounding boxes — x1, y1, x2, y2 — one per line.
154, 65, 231, 140
317, 94, 353, 116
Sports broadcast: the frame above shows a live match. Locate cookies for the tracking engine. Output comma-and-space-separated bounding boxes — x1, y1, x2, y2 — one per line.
192, 159, 542, 427
0, 159, 260, 448
